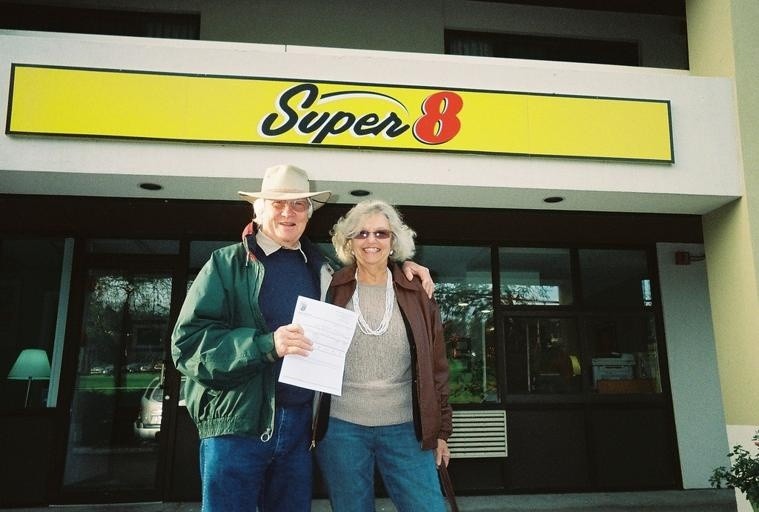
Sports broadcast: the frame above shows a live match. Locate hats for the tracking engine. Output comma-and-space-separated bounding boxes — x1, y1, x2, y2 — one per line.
236, 163, 333, 214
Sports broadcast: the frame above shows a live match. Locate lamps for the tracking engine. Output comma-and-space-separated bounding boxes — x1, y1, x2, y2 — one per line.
6, 348, 51, 408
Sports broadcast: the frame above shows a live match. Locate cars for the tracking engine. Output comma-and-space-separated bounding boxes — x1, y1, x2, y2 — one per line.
86, 361, 165, 376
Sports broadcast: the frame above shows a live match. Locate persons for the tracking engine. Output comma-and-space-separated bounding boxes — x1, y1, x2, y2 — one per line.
318, 200, 454, 511
170, 163, 437, 512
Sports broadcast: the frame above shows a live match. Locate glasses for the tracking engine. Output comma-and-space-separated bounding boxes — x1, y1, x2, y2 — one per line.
351, 228, 394, 240
261, 199, 311, 211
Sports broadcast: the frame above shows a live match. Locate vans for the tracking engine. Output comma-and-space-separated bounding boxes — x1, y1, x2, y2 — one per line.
132, 374, 191, 464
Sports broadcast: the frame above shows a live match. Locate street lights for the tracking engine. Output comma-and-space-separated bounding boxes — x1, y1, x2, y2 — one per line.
479, 307, 492, 395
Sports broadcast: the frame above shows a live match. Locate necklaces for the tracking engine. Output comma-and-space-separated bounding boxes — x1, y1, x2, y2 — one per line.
351, 267, 396, 336
